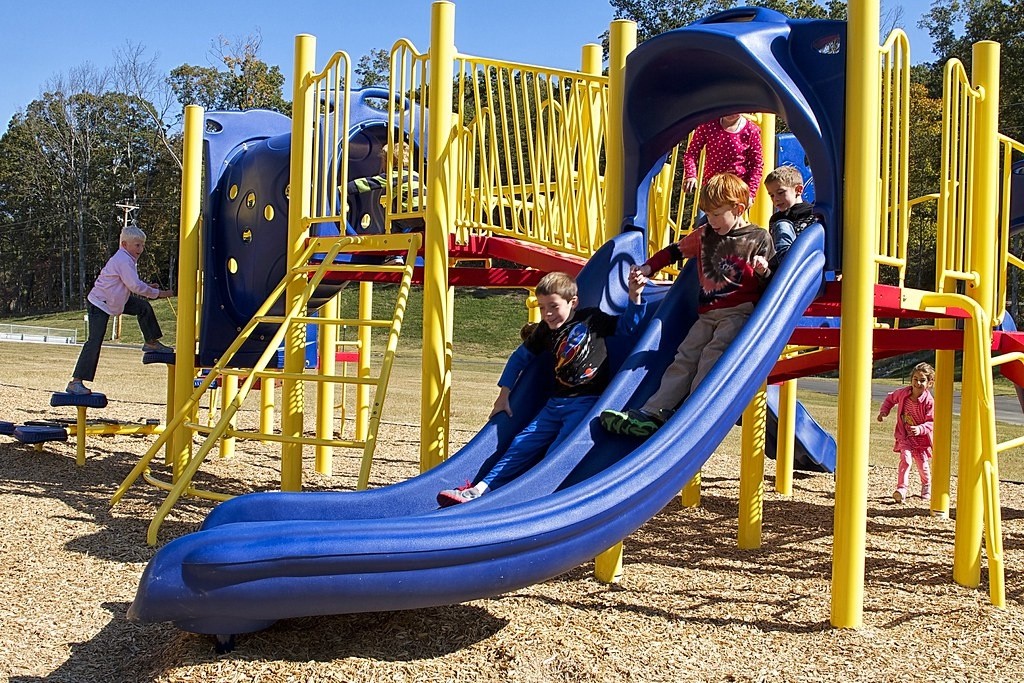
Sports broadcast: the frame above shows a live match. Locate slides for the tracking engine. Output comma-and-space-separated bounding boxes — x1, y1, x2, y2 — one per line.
736, 379, 837, 476
126, 224, 828, 634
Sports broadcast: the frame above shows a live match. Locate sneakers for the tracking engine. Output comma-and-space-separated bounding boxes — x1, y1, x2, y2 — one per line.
65, 380, 92, 396
921, 484, 931, 502
436, 480, 491, 508
893, 488, 907, 505
142, 340, 174, 353
599, 410, 665, 446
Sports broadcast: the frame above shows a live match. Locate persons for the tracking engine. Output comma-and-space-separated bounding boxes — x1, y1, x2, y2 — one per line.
65, 225, 174, 393
336, 143, 426, 265
600, 175, 779, 441
684, 113, 765, 225
766, 167, 819, 252
435, 267, 649, 508
877, 361, 935, 502
